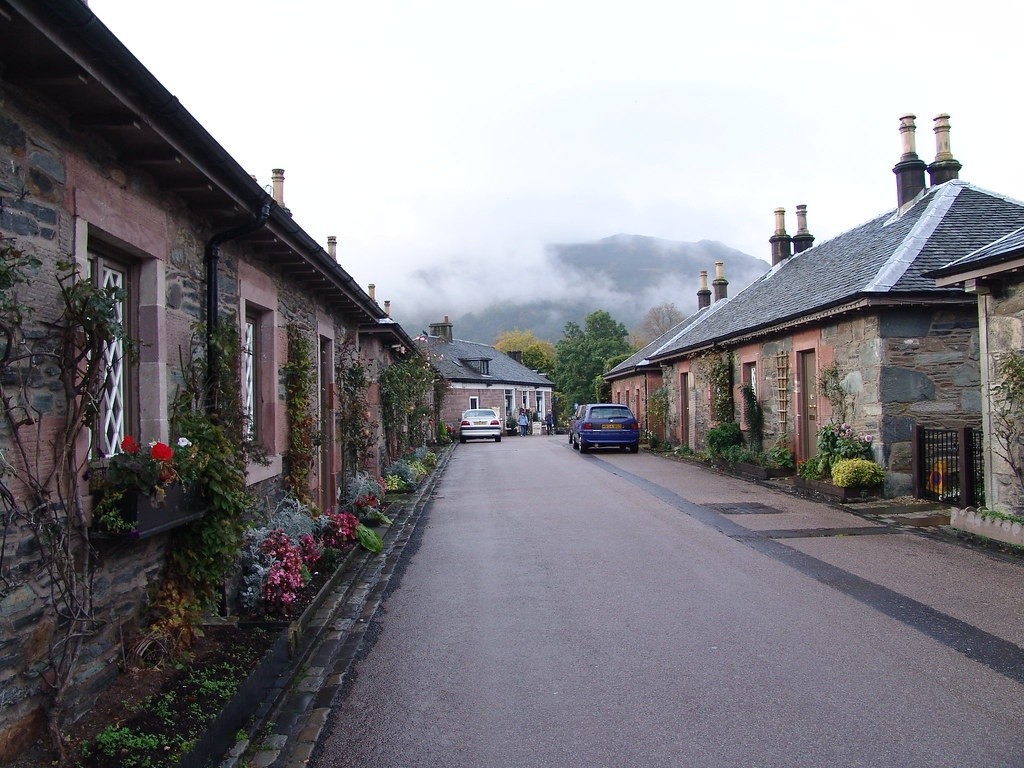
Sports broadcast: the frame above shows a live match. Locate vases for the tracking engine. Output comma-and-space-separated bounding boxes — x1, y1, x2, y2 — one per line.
795, 474, 886, 497
95, 464, 208, 542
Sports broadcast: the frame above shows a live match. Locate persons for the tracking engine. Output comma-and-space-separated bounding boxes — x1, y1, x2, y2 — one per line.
517, 408, 531, 437
545, 412, 554, 436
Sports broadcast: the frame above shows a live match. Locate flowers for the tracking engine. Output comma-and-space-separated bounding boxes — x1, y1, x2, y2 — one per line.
800, 421, 886, 486
84, 423, 198, 544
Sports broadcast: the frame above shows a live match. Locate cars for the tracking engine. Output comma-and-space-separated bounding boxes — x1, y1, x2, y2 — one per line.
458, 408, 503, 444
567, 404, 586, 444
571, 403, 640, 453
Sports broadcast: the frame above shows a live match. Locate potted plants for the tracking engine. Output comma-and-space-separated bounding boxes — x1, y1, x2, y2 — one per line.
702, 349, 793, 479
